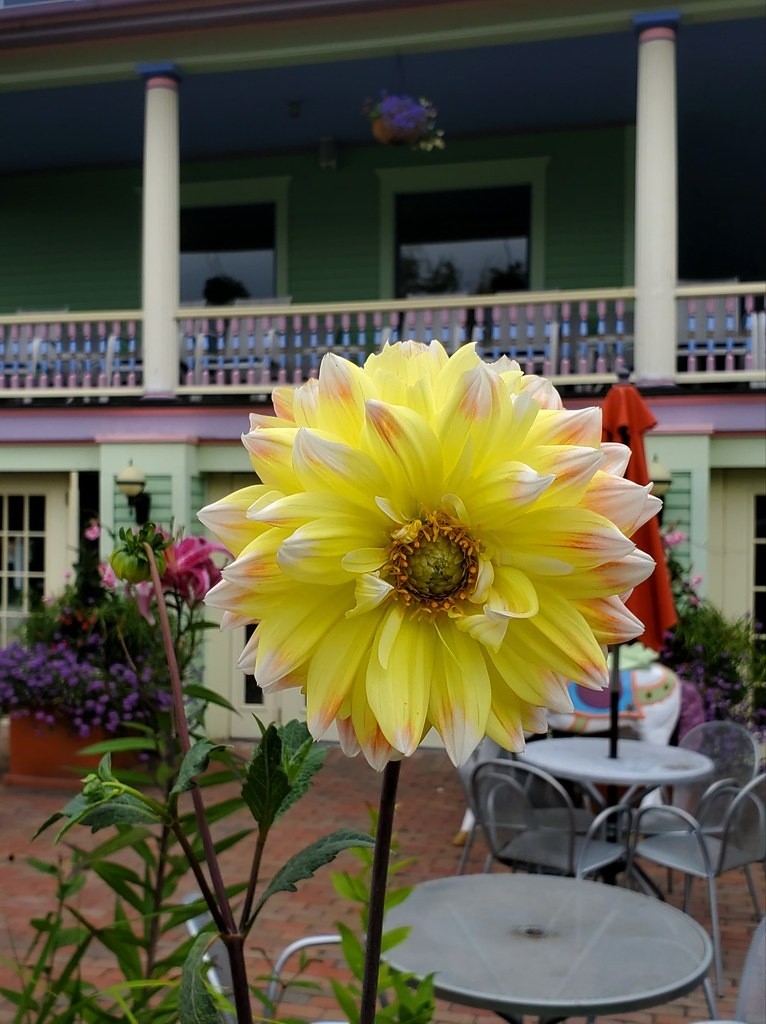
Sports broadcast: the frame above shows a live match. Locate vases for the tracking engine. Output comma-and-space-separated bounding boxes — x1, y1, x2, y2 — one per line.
5, 709, 177, 789
371, 117, 420, 143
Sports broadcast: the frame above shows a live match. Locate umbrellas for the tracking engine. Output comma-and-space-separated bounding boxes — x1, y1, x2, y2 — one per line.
599, 382, 678, 888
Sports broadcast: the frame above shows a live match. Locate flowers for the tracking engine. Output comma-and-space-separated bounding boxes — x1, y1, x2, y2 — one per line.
360, 97, 444, 151
0, 519, 209, 762
1, 340, 663, 1023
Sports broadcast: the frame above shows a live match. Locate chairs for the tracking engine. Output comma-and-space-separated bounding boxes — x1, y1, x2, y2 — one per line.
461, 718, 765, 1024
180, 892, 351, 1024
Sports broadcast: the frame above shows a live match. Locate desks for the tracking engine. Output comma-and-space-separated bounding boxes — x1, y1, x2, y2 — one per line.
517, 737, 714, 900
359, 873, 714, 1024
